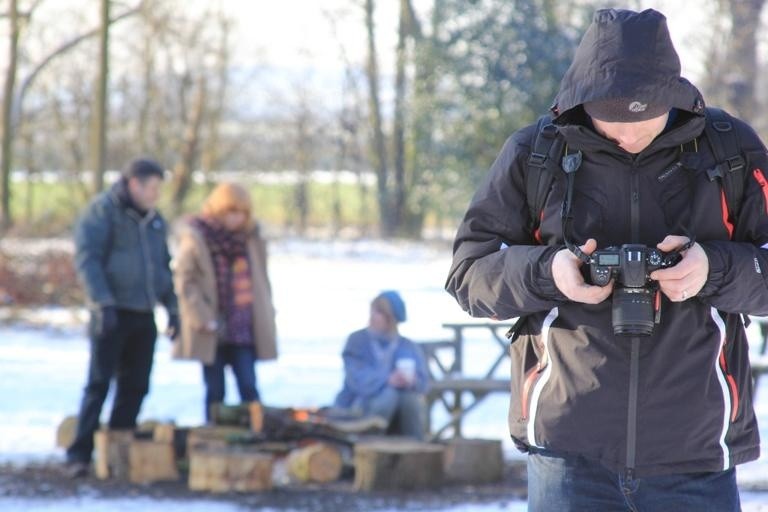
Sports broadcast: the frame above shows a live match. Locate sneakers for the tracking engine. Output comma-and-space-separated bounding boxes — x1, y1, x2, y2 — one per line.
109, 416, 140, 432
66, 437, 94, 454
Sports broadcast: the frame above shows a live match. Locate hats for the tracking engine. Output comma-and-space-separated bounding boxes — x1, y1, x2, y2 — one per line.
383, 290, 407, 322
583, 97, 673, 122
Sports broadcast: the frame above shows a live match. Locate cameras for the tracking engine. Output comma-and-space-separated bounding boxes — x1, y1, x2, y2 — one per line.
588, 242, 683, 338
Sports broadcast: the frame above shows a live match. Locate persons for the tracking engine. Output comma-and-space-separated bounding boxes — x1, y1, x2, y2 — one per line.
59, 156, 181, 479
443, 7, 767, 511
167, 179, 278, 434
330, 288, 432, 439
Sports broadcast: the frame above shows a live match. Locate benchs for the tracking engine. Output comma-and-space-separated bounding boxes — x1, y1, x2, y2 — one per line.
416, 339, 511, 442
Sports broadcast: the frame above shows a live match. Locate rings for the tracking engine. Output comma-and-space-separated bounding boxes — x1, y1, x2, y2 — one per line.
683, 290, 687, 301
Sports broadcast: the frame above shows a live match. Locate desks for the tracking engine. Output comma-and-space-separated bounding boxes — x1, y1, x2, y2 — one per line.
442, 311, 515, 441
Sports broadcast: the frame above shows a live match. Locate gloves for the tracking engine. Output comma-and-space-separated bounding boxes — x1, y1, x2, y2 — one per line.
169, 307, 180, 340
98, 307, 119, 335
